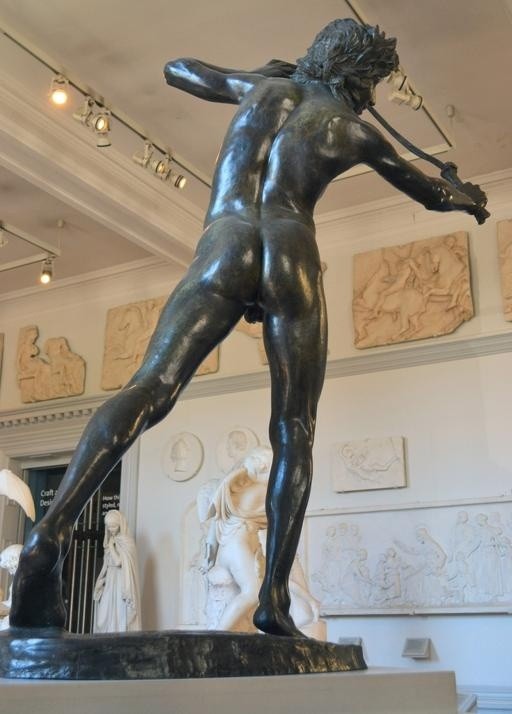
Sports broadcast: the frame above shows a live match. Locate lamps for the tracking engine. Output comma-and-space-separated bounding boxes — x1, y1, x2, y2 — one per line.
39, 258, 53, 285
384, 73, 423, 111
50, 73, 187, 190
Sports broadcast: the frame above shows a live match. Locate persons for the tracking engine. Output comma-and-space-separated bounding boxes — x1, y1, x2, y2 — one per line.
8, 17, 492, 645
92, 507, 142, 633
0, 542, 23, 632
192, 420, 324, 636
